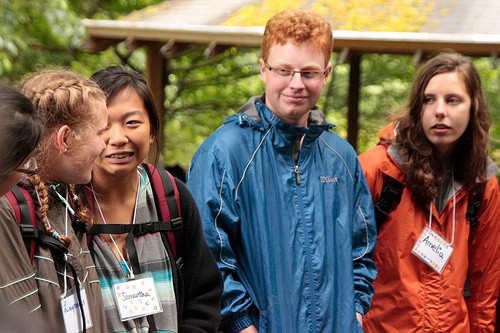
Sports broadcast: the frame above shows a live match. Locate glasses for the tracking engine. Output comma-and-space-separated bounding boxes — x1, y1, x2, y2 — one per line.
265, 60, 328, 82
14, 156, 38, 176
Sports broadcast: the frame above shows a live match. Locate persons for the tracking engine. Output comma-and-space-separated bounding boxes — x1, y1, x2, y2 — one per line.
0, 70, 109, 333
0, 83, 41, 196
77, 64, 225, 333
184, 9, 379, 333
356, 53, 500, 333
